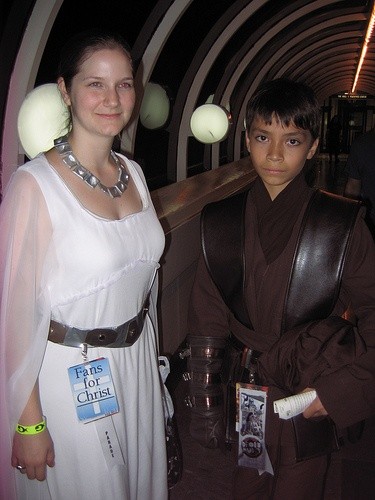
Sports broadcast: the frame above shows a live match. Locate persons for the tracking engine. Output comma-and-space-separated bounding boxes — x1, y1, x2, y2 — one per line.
325, 115, 343, 163
0, 26, 174, 500
345, 127, 375, 241
188, 78, 375, 500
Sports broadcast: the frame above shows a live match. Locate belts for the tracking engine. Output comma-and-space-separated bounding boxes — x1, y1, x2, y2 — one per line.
48, 291, 153, 349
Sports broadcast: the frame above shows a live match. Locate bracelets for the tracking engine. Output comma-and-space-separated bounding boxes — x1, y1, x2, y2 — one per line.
16, 416, 46, 435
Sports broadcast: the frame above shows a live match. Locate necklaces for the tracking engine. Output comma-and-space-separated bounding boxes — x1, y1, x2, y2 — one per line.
54, 136, 129, 199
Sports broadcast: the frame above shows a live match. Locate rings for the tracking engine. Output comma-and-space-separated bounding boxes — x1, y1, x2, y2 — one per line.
17, 465, 24, 469
